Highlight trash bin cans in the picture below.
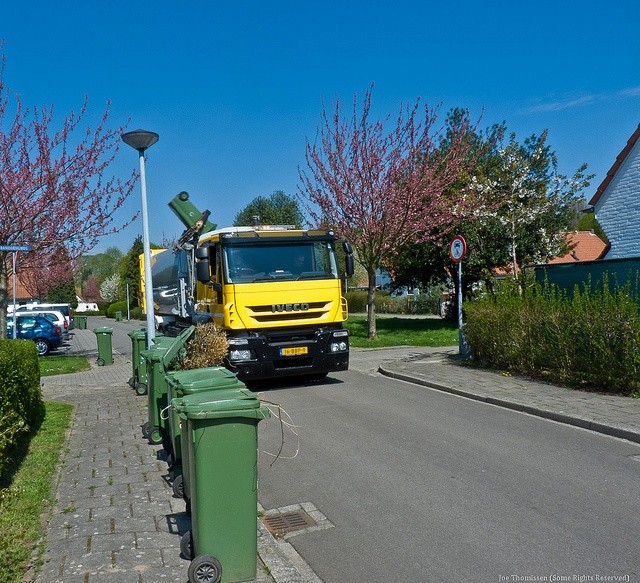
[139,344,169,444]
[73,316,80,328]
[169,387,271,583]
[115,311,122,322]
[127,328,165,394]
[79,316,87,329]
[163,364,246,497]
[167,191,217,236]
[152,336,190,424]
[161,325,220,448]
[93,326,114,365]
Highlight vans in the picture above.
[12,303,73,330]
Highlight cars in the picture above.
[6,316,63,356]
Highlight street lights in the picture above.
[122,128,159,349]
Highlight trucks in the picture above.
[138,216,354,389]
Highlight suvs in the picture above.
[7,311,68,350]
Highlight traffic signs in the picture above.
[0,245,30,251]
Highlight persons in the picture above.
[287,253,307,275]
